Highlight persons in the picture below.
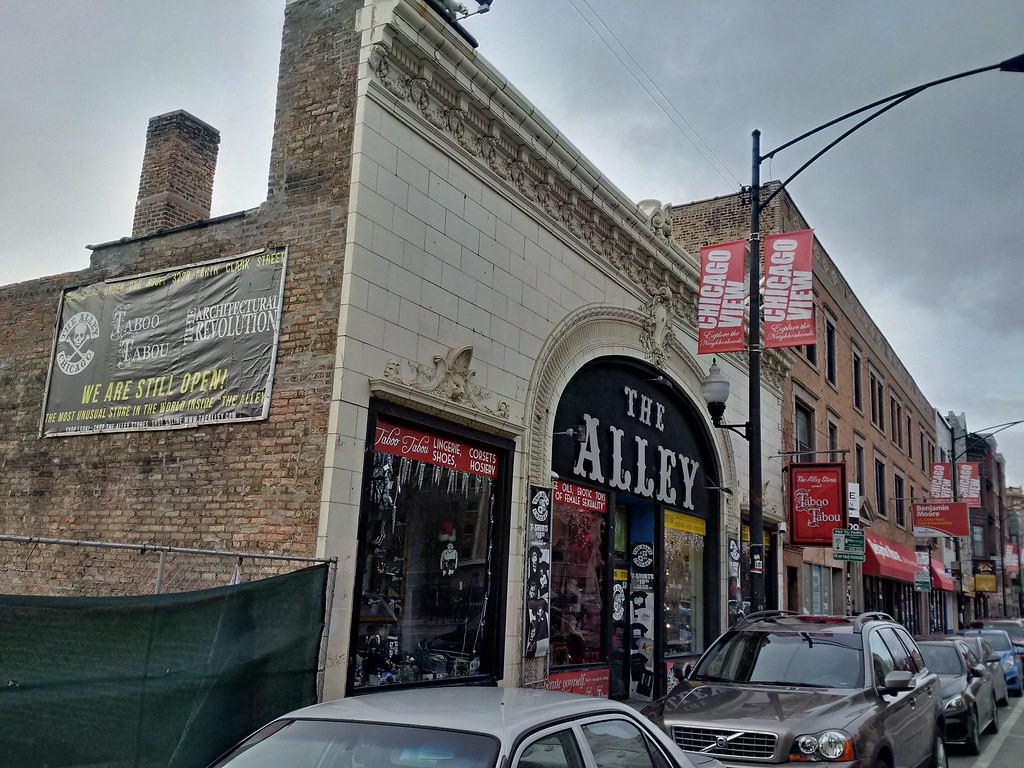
[550,577,587,665]
[728,576,737,600]
[526,545,548,657]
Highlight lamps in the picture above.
[552,425,586,442]
[705,487,734,498]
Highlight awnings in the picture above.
[931,562,954,592]
[864,531,925,583]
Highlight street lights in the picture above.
[700,48,1024,611]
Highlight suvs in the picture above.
[635,610,951,768]
[960,616,1024,664]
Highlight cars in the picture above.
[911,637,1000,757]
[923,634,1010,709]
[955,628,1024,698]
[205,683,720,767]
